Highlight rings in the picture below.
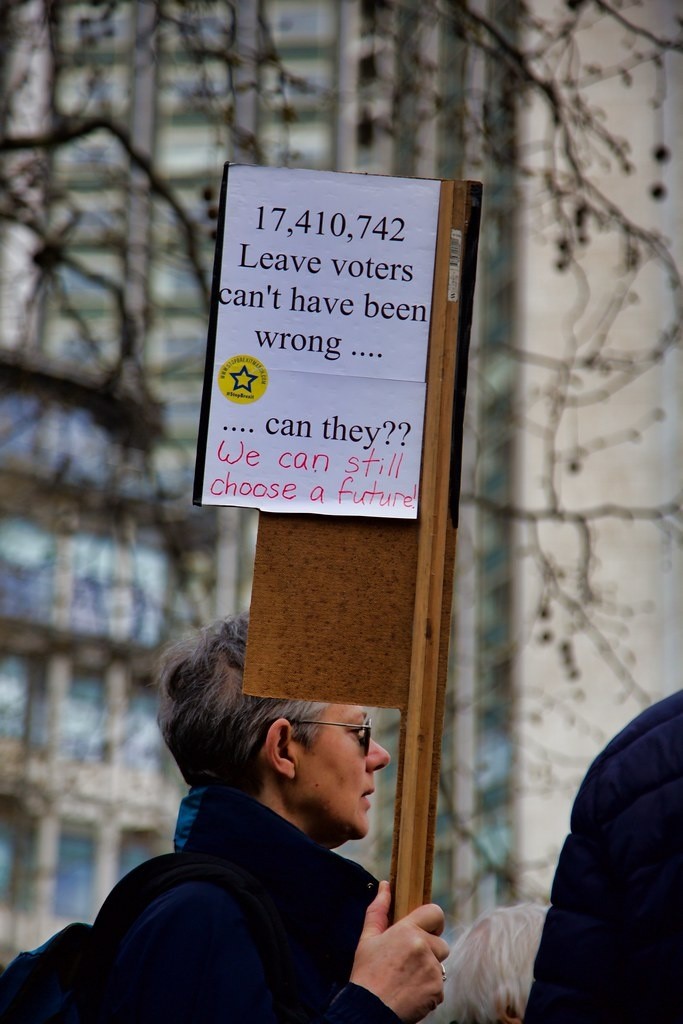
[441,963,446,981]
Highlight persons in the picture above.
[66,618,450,1024]
[442,903,552,1024]
[519,689,683,1024]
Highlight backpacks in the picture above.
[0,851,301,1024]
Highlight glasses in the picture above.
[249,716,373,770]
